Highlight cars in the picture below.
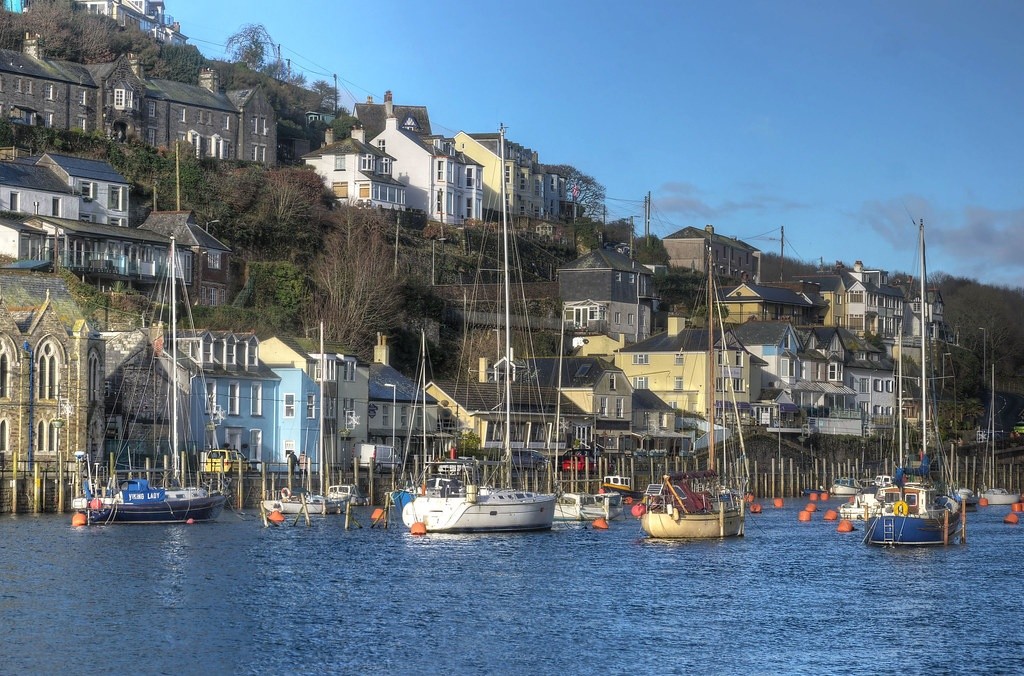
[562,455,599,474]
[203,450,249,472]
[501,449,550,471]
[551,449,594,471]
[438,459,478,477]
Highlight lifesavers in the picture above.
[893,500,909,516]
[281,486,291,499]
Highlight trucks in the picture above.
[350,442,404,473]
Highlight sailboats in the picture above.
[640,246,749,538]
[390,327,466,511]
[71,235,231,526]
[540,312,625,521]
[261,317,349,513]
[863,217,965,547]
[976,362,1019,504]
[401,120,557,535]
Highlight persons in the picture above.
[298,451,309,479]
[287,451,298,476]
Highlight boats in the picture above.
[831,475,979,520]
[327,483,366,507]
